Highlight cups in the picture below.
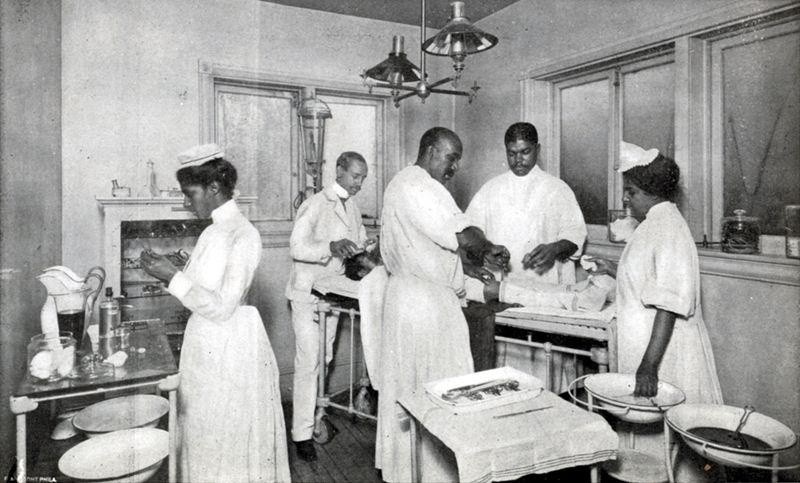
[50,291,88,358]
[783,205,800,260]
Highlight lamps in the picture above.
[360,1,499,109]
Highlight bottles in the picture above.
[25,330,77,384]
[608,209,640,245]
[98,287,122,341]
[720,209,762,255]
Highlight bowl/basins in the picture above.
[58,427,172,483]
[51,417,79,441]
[582,372,687,422]
[666,403,797,468]
[71,393,174,438]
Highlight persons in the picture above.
[466,122,588,397]
[341,243,608,312]
[284,151,369,464]
[375,125,509,482]
[581,141,727,419]
[138,140,292,483]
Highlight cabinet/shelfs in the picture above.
[97,194,256,351]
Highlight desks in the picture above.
[8,320,182,481]
[396,369,616,482]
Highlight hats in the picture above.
[176,144,223,171]
[618,141,659,173]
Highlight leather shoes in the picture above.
[293,439,316,462]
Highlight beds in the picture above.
[311,274,618,446]
